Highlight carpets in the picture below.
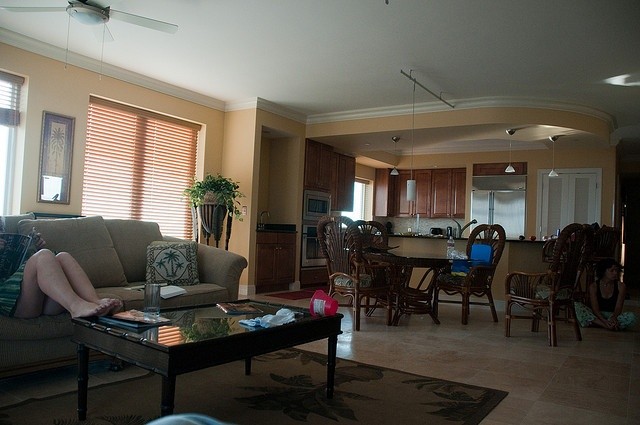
[0,346,510,424]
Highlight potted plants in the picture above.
[179,171,244,223]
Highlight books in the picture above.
[0,231,49,280]
[216,301,264,315]
[106,309,172,323]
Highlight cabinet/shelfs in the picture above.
[431,169,465,218]
[331,151,356,211]
[394,169,431,218]
[300,270,328,288]
[256,232,297,287]
[375,167,396,218]
[304,138,334,191]
[536,167,603,242]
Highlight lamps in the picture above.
[504,165,516,173]
[390,167,400,176]
[549,170,559,176]
[406,179,416,202]
[73,11,101,27]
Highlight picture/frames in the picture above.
[35,108,77,205]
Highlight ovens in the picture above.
[300,224,327,271]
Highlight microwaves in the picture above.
[303,189,332,221]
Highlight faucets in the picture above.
[453,220,477,238]
[258,211,270,231]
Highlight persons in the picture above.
[0,216,124,322]
[572,257,639,332]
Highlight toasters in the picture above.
[429,228,443,237]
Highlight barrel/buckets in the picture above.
[309,290,339,317]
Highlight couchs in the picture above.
[0,236,250,381]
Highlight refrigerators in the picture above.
[470,190,527,240]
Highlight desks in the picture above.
[398,254,463,294]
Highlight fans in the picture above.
[0,0,178,35]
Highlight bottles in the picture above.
[447,236,455,259]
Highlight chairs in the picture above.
[344,218,397,319]
[363,248,442,326]
[503,222,590,348]
[593,224,620,259]
[541,223,592,263]
[432,222,501,326]
[316,214,393,331]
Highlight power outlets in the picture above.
[408,228,412,232]
[242,206,247,215]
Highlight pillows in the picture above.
[104,218,163,282]
[17,215,129,289]
[0,212,36,235]
[145,239,200,286]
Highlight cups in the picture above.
[144,284,160,316]
